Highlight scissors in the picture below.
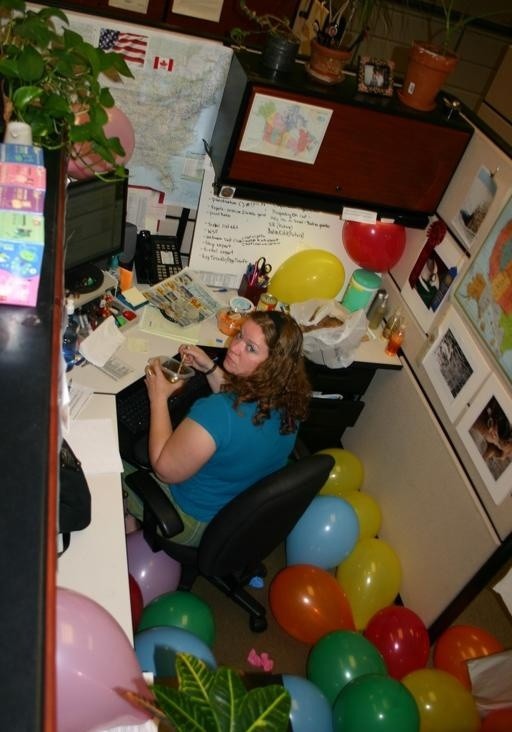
[257,257,271,274]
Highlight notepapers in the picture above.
[121,287,148,306]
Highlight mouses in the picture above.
[206,351,219,362]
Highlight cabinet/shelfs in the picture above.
[205,42,475,232]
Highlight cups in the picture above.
[3,119,33,145]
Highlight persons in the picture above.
[121,310,313,549]
[369,71,384,88]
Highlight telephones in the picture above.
[135,230,182,283]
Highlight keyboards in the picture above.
[116,369,204,435]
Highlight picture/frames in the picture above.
[448,180,512,397]
[418,302,493,428]
[355,54,398,95]
[455,369,512,508]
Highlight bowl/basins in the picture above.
[65,262,105,294]
[215,307,248,337]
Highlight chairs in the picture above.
[123,415,337,634]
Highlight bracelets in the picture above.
[204,362,217,376]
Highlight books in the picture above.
[139,305,201,346]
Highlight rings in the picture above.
[147,370,153,376]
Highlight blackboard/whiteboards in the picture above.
[189,169,382,304]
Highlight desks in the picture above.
[45,260,402,465]
[54,381,157,732]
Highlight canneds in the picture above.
[256,292,278,311]
[216,297,255,337]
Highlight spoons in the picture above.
[170,347,193,385]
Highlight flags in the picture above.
[98,27,149,67]
[152,56,173,72]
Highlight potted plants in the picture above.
[301,0,394,87]
[395,0,510,113]
[226,0,302,87]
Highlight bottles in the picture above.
[383,306,406,356]
[341,269,382,315]
[366,289,389,331]
[62,304,78,374]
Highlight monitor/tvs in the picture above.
[64,167,129,294]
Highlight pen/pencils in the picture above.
[247,263,271,287]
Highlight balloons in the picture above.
[282,605,479,732]
[269,446,402,643]
[55,586,157,732]
[341,219,406,273]
[67,101,136,181]
[127,530,216,688]
[267,248,346,306]
[436,623,512,732]
